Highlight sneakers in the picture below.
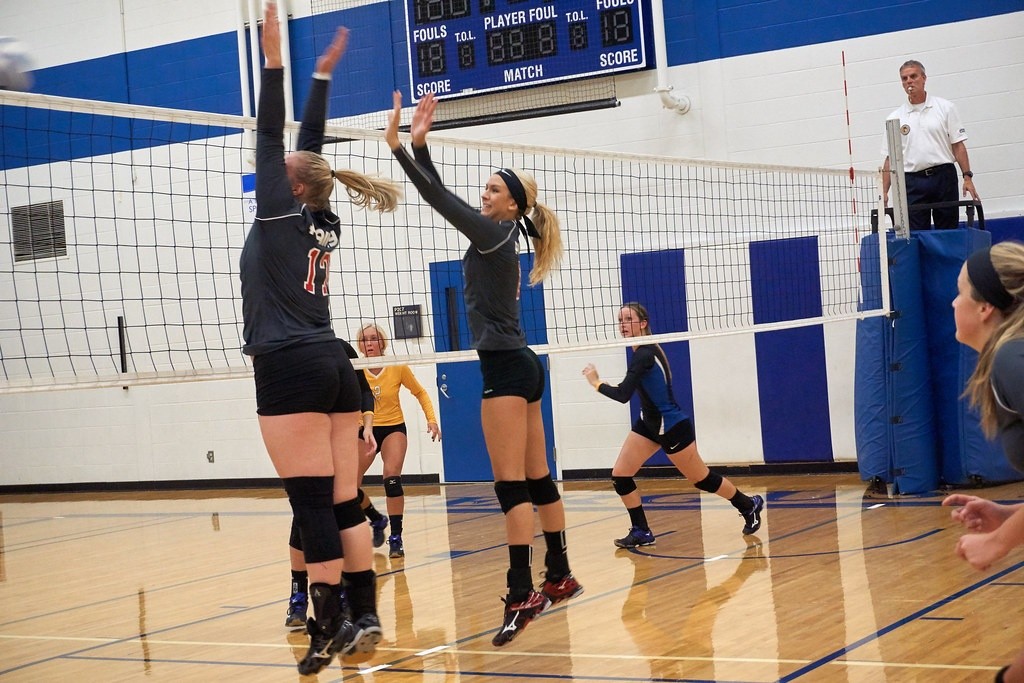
[284,592,309,627]
[347,613,384,656]
[491,588,553,648]
[370,515,388,548]
[614,526,657,549]
[296,614,365,677]
[740,494,764,536]
[534,569,585,609]
[385,534,405,558]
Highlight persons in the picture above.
[942,242,1024,683]
[284,337,377,627]
[239,0,384,676]
[385,90,585,646]
[357,323,442,558]
[882,60,981,231]
[582,302,764,548]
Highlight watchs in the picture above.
[963,171,973,178]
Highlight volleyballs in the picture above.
[0,36,35,91]
